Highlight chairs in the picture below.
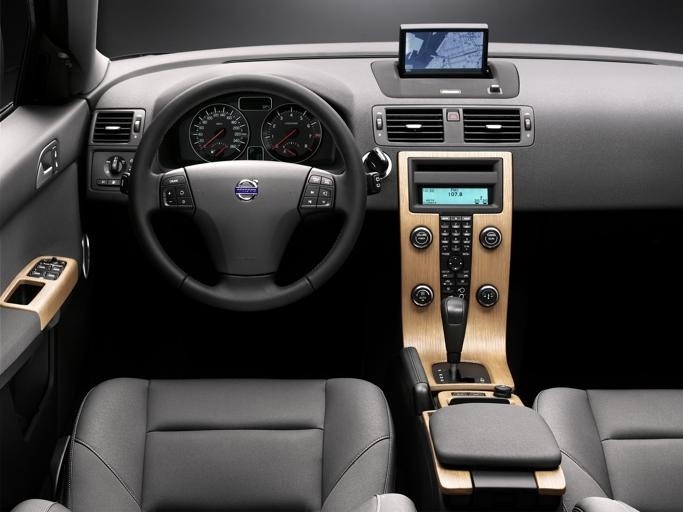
[532,386,682,511]
[8,376,417,512]
